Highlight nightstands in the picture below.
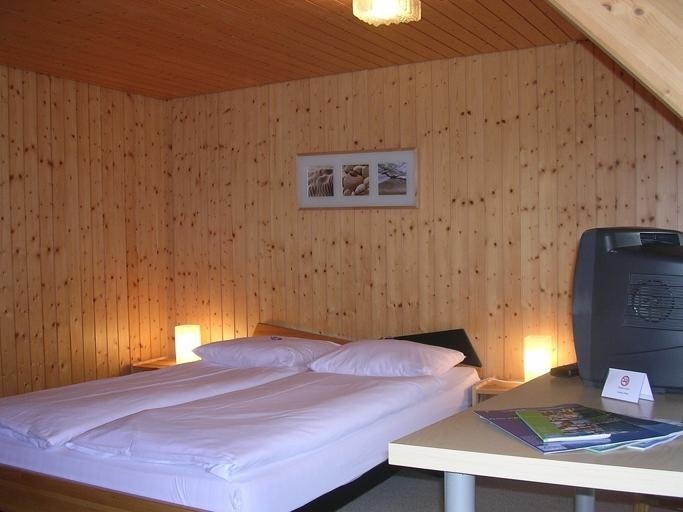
[470,376,523,407]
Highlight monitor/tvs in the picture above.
[572,226,683,395]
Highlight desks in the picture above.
[387,365,682,512]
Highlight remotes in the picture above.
[551,363,579,378]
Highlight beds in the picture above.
[0,322,480,512]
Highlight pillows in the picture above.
[190,334,465,378]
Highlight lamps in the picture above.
[174,324,200,365]
[353,0,421,28]
[524,335,551,383]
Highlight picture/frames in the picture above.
[294,146,418,209]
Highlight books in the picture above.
[472,403,683,456]
